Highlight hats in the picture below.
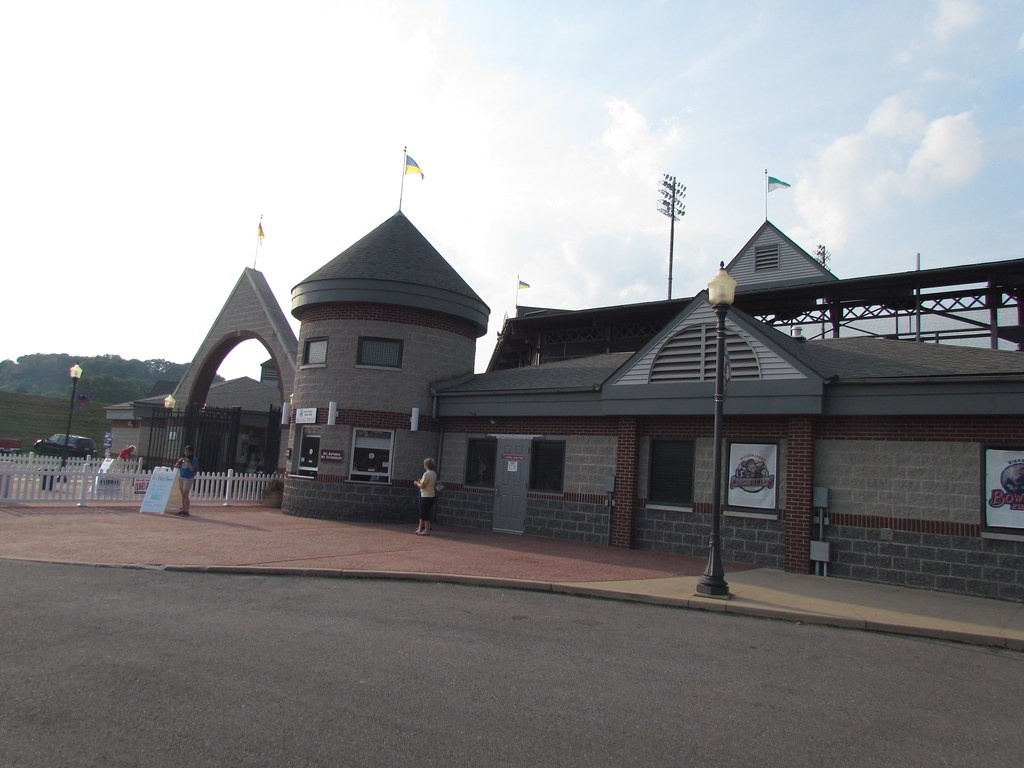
[185,446,193,452]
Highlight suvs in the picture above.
[33,433,98,462]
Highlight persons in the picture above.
[175,445,199,517]
[254,461,264,475]
[414,457,439,537]
[117,445,136,463]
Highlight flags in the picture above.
[518,281,530,289]
[768,177,790,192]
[258,223,265,246]
[405,155,425,180]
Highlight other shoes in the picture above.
[418,532,430,536]
[414,527,424,531]
[422,527,432,530]
[414,530,420,534]
[176,511,184,515]
[181,512,189,517]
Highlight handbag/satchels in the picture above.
[435,479,445,492]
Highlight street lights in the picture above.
[693,260,736,601]
[159,392,177,467]
[54,360,83,483]
